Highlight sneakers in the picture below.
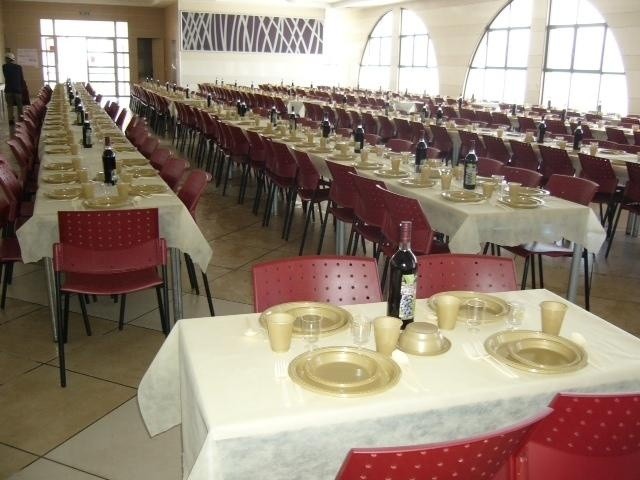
[9,120,15,125]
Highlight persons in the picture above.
[2,52,30,126]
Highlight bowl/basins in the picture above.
[401,322,444,351]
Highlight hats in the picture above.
[3,53,16,61]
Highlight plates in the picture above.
[509,339,580,366]
[285,306,343,331]
[373,170,408,178]
[400,336,450,356]
[136,184,166,194]
[290,346,400,396]
[46,84,65,121]
[45,133,66,137]
[133,168,158,176]
[499,195,544,208]
[601,149,624,154]
[486,331,586,373]
[351,162,383,169]
[43,162,71,169]
[45,121,62,125]
[259,301,352,338]
[427,291,509,320]
[125,158,150,165]
[297,142,317,147]
[92,196,126,207]
[44,149,68,153]
[442,190,485,202]
[42,174,77,183]
[310,148,335,153]
[613,160,626,165]
[45,189,82,198]
[43,126,63,130]
[117,147,134,151]
[306,351,378,388]
[522,187,550,196]
[44,140,66,144]
[400,178,435,187]
[329,154,356,160]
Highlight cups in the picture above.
[450,120,455,128]
[596,122,602,128]
[64,82,74,144]
[116,183,129,198]
[71,144,79,155]
[590,142,598,146]
[401,152,410,163]
[468,299,484,327]
[590,146,597,156]
[360,149,369,162]
[116,160,122,171]
[441,175,451,190]
[375,145,385,157]
[122,174,131,182]
[307,134,313,143]
[483,183,495,199]
[436,295,457,328]
[373,316,403,355]
[493,175,503,191]
[227,112,230,119]
[510,303,522,324]
[78,170,87,182]
[349,315,370,344]
[390,156,401,175]
[256,118,260,125]
[508,182,522,199]
[560,140,566,149]
[266,313,292,350]
[525,132,532,143]
[540,300,567,335]
[71,158,80,169]
[301,314,320,341]
[472,123,480,130]
[497,129,503,138]
[220,84,300,101]
[82,182,94,197]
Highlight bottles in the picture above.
[216,77,314,91]
[418,88,603,121]
[241,100,245,116]
[435,103,443,125]
[388,220,416,328]
[237,97,241,114]
[323,112,331,136]
[421,106,427,124]
[538,115,546,143]
[464,141,476,188]
[146,76,212,107]
[354,114,365,153]
[289,106,296,135]
[271,99,277,126]
[103,136,117,184]
[84,111,93,148]
[333,84,409,98]
[416,130,428,173]
[67,79,86,124]
[573,121,582,151]
